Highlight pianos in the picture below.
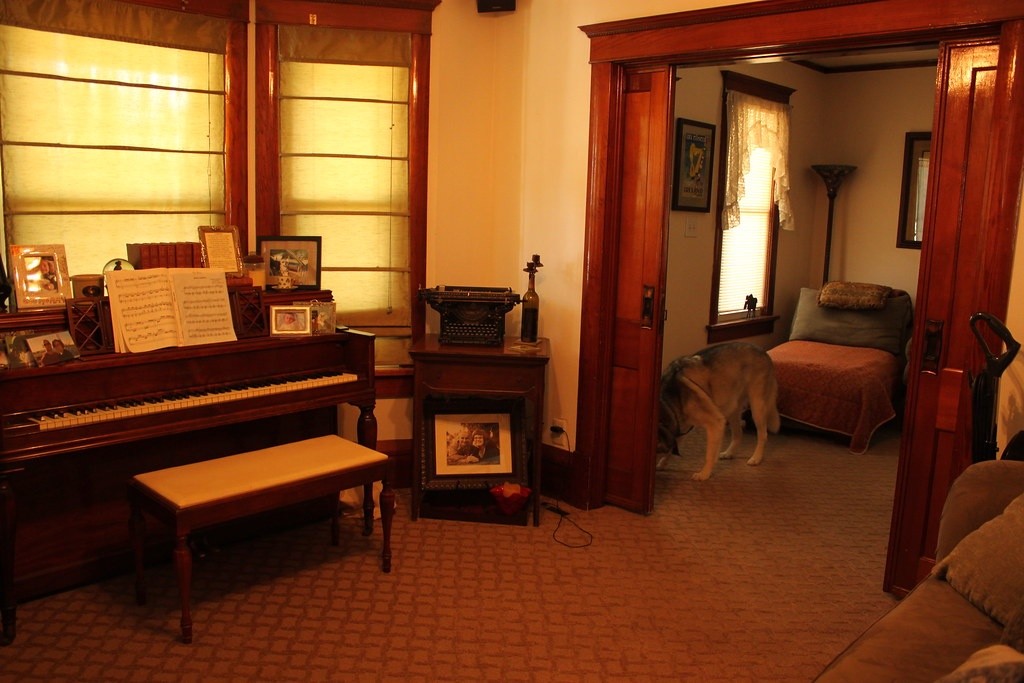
[2,285,378,645]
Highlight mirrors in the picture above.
[895,130,932,249]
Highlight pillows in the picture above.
[932,490,1024,631]
[818,281,888,312]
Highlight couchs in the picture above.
[808,461,1024,683]
[751,285,913,455]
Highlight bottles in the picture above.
[521,275,539,343]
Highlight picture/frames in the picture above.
[673,117,717,213]
[0,226,513,475]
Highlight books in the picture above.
[105,267,237,353]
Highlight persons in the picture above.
[280,313,301,330]
[40,339,74,367]
[40,258,56,289]
[9,341,34,365]
[448,429,498,463]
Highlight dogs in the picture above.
[657,341,780,481]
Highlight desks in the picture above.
[408,333,552,527]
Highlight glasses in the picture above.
[44,342,50,346]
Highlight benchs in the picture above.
[132,436,394,643]
